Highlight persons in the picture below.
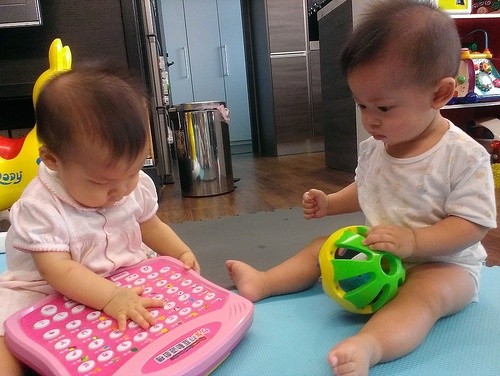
[1,57,202,376]
[218,0,497,375]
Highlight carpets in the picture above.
[205,264,500,376]
[167,204,372,292]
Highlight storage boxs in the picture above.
[420,0,472,14]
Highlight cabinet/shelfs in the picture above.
[251,0,324,157]
[442,12,500,109]
[160,0,254,154]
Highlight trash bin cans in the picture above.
[175,101,241,199]
